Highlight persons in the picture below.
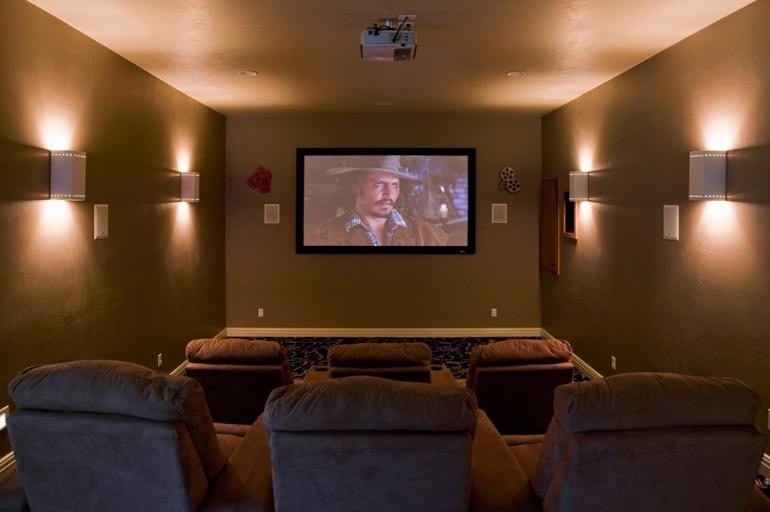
[309,155,449,246]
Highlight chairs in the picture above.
[504,372,769,511]
[465,337,574,435]
[6,360,274,511]
[327,342,432,379]
[185,338,301,424]
[264,363,477,510]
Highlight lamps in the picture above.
[688,151,728,200]
[49,151,86,203]
[180,172,201,202]
[568,171,588,201]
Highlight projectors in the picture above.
[360,31,418,61]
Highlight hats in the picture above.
[326,156,423,186]
